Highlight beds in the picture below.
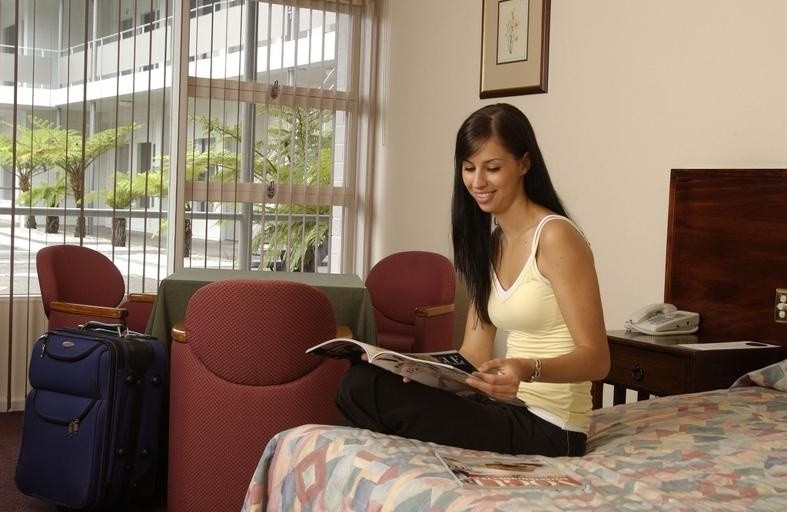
[240,170,785,512]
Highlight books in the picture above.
[305,337,483,394]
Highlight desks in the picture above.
[143,268,377,344]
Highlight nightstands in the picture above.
[589,327,780,409]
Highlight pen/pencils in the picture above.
[746,343,768,346]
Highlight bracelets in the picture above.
[525,357,541,382]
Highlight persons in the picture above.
[336,103,612,456]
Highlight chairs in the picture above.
[166,279,354,510]
[363,250,455,352]
[36,245,158,335]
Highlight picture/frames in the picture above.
[477,1,549,100]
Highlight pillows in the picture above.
[728,359,785,392]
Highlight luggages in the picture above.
[14,320,169,512]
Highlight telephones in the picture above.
[624,302,700,336]
